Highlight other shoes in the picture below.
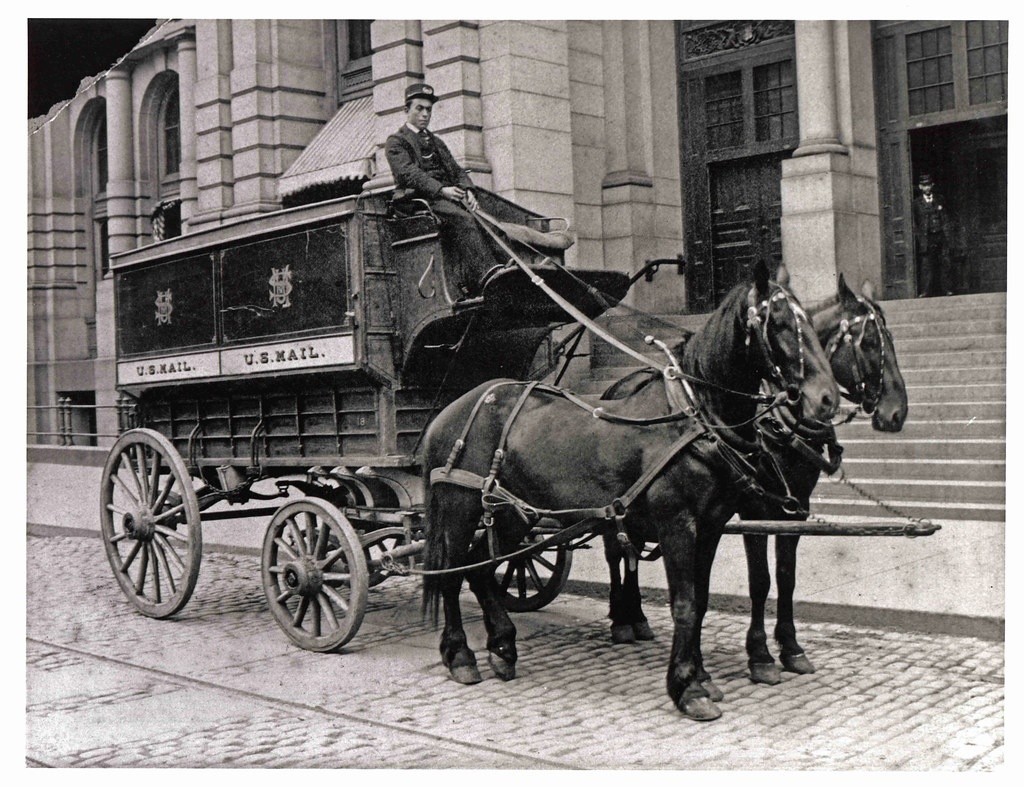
[919,292,931,298]
[946,291,954,296]
[478,263,505,288]
[532,255,562,267]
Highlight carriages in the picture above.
[96,183,947,727]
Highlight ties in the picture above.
[419,130,427,138]
[927,197,932,206]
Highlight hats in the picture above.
[918,174,933,185]
[405,84,439,104]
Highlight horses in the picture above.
[420,260,908,721]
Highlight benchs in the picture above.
[385,187,570,345]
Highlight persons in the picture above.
[911,173,958,299]
[383,82,517,297]
